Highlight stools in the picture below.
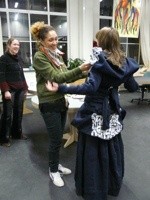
[131,83,150,105]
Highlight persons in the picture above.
[31,21,91,187]
[45,26,140,200]
[0,38,28,146]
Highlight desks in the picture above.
[31,95,87,149]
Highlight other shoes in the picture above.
[7,139,13,146]
[13,134,28,139]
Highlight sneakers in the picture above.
[49,167,65,186]
[58,163,71,174]
